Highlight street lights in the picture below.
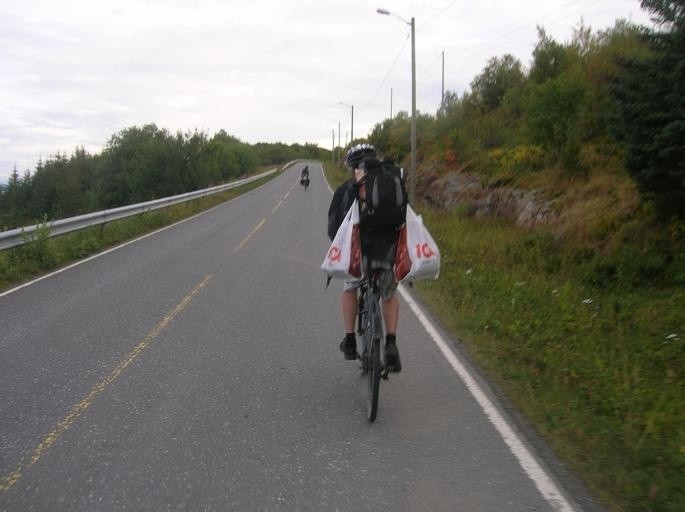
[338,102,354,147]
[377,9,417,208]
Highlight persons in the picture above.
[327,142,412,375]
[301,164,309,183]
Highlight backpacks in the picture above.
[355,172,407,262]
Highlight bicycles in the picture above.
[300,174,310,191]
[344,264,401,423]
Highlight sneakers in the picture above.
[339,333,356,360]
[383,335,401,374]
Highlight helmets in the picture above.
[346,143,375,166]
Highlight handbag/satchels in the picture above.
[393,203,441,284]
[320,199,364,282]
